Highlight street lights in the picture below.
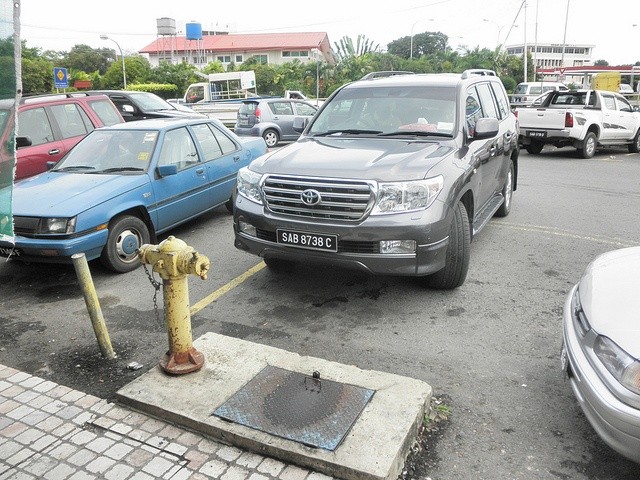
[99,33,131,91]
[480,14,518,61]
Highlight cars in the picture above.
[0,117,263,274]
[561,244,640,466]
[234,98,318,149]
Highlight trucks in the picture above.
[183,82,247,104]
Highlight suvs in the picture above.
[233,68,520,291]
[79,87,211,122]
[0,95,128,191]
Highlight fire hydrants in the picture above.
[134,235,211,375]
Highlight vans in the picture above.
[512,81,570,108]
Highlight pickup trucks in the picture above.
[517,90,640,158]
